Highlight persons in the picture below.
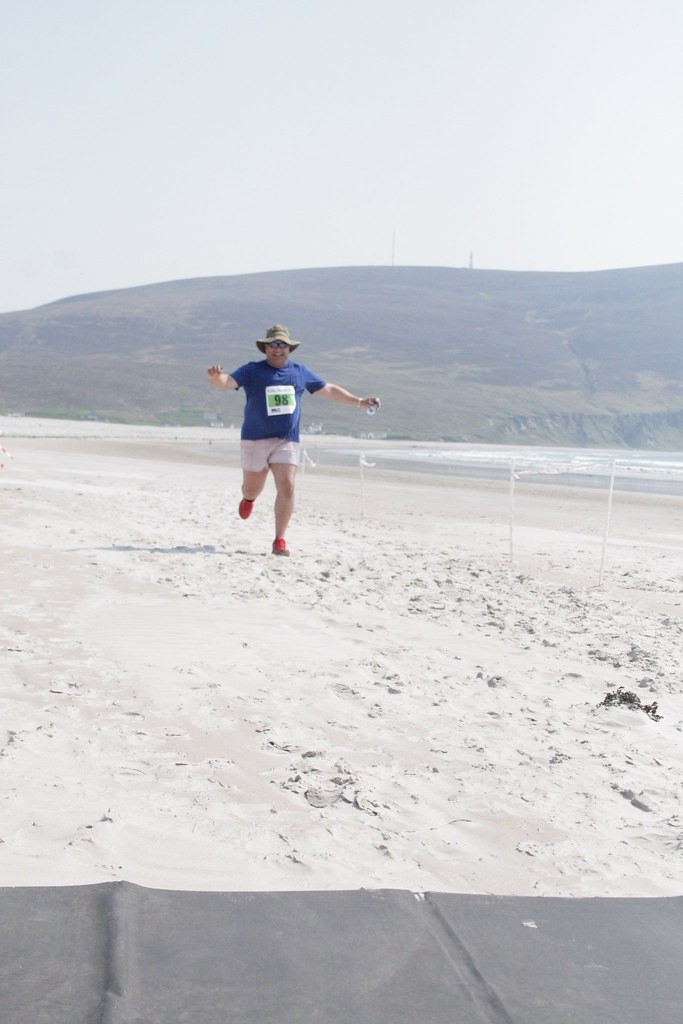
[208,325,381,556]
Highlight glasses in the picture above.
[266,342,289,349]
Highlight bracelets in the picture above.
[357,397,363,408]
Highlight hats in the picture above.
[256,325,301,353]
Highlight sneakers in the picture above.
[238,498,254,519]
[272,536,290,556]
